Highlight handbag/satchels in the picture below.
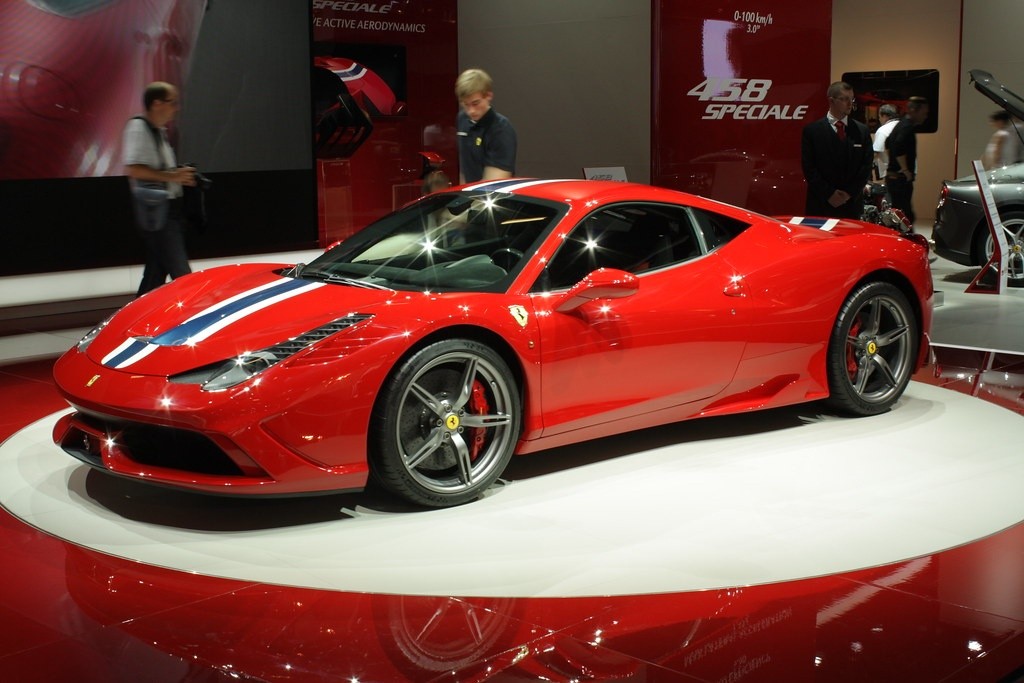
[131,186,170,232]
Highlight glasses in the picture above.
[834,97,856,102]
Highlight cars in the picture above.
[929,67,1024,285]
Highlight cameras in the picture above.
[178,162,212,189]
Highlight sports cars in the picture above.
[50,176,935,509]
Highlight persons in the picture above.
[979,109,1024,171]
[453,68,518,244]
[798,81,873,221]
[122,81,195,299]
[871,103,919,233]
[421,170,449,195]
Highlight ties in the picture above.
[835,121,845,141]
